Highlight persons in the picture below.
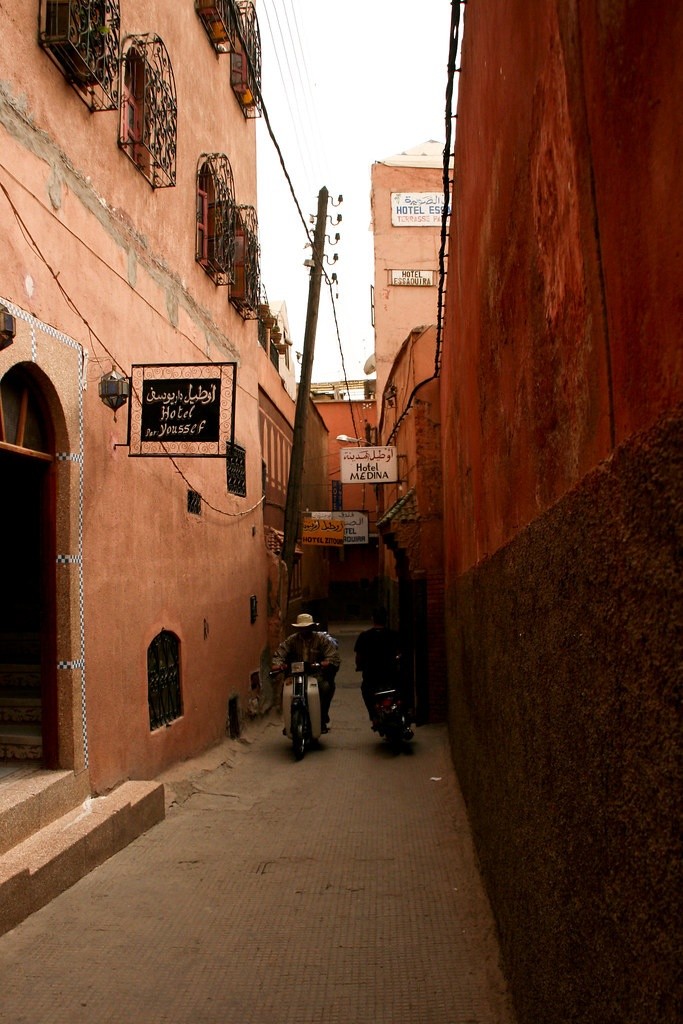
[354,606,416,732]
[272,613,342,736]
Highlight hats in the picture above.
[291,613,320,627]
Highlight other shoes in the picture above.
[321,722,328,735]
[283,727,288,735]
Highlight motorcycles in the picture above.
[356,657,415,755]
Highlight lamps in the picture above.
[98,364,133,450]
[336,434,375,447]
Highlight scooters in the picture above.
[264,660,338,761]
